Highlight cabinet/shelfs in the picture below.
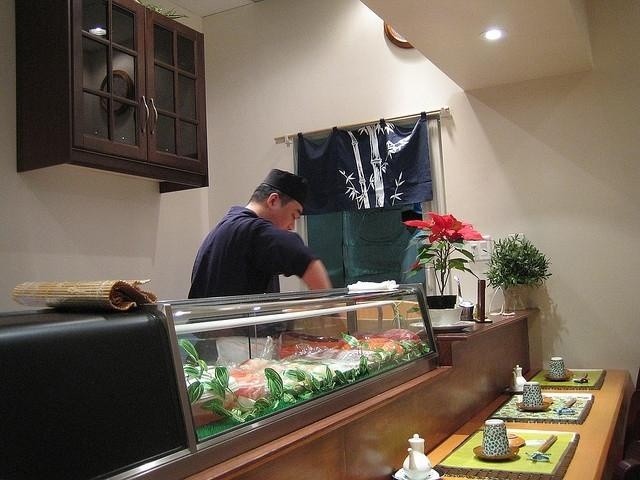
[16,0,209,194]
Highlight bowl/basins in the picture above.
[426,306,462,326]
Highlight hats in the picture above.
[264,169,309,207]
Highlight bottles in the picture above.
[459,301,475,320]
[408,433,425,452]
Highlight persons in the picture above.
[179,168,347,365]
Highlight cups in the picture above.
[482,419,510,456]
[549,358,565,379]
[522,383,544,407]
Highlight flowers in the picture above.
[401,211,486,294]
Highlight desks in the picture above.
[389,367,633,480]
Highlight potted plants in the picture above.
[482,232,554,312]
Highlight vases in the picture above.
[426,295,457,310]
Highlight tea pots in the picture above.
[511,365,528,392]
[403,448,432,478]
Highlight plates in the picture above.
[546,370,574,381]
[516,401,550,411]
[393,466,440,480]
[473,445,519,460]
[409,319,476,334]
